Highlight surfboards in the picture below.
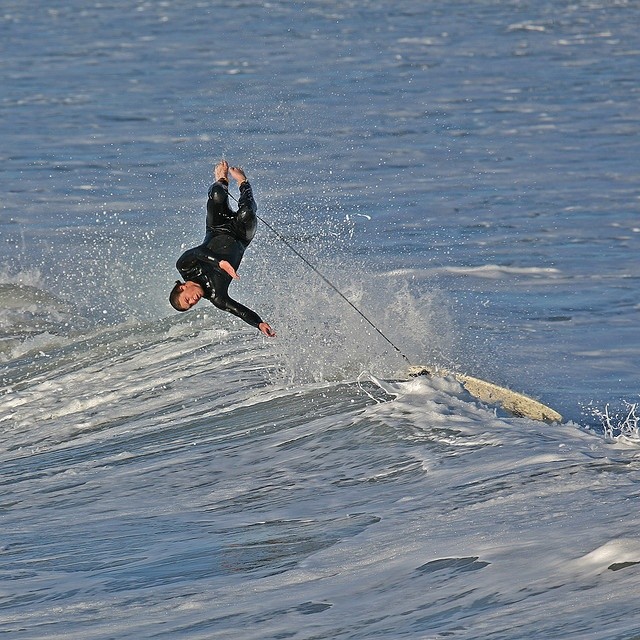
[405,364,563,430]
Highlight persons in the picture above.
[169,161,275,337]
